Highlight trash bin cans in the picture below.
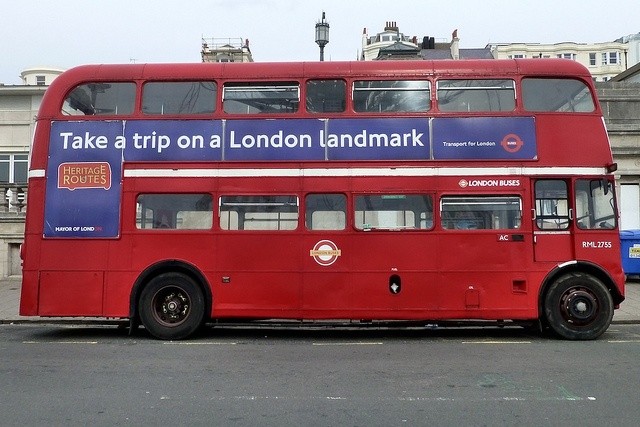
[620,230,640,280]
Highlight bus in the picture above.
[19,59,625,339]
